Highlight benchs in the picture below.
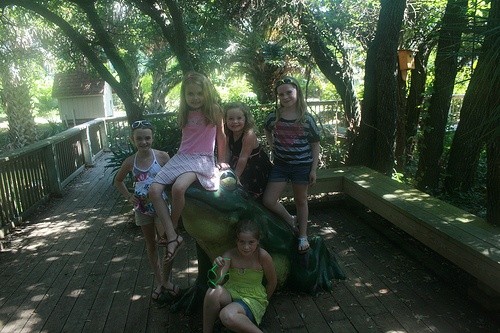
[342,164,500,290]
[281,166,349,195]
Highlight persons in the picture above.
[202,218,277,333]
[224,102,273,203]
[262,76,320,251]
[147,72,226,261]
[115,120,181,303]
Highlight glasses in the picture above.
[132,120,152,129]
[275,78,292,87]
[206,257,231,289]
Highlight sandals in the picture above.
[161,282,182,300]
[164,233,185,264]
[290,215,299,237]
[296,234,311,254]
[151,286,163,302]
[158,232,167,245]
